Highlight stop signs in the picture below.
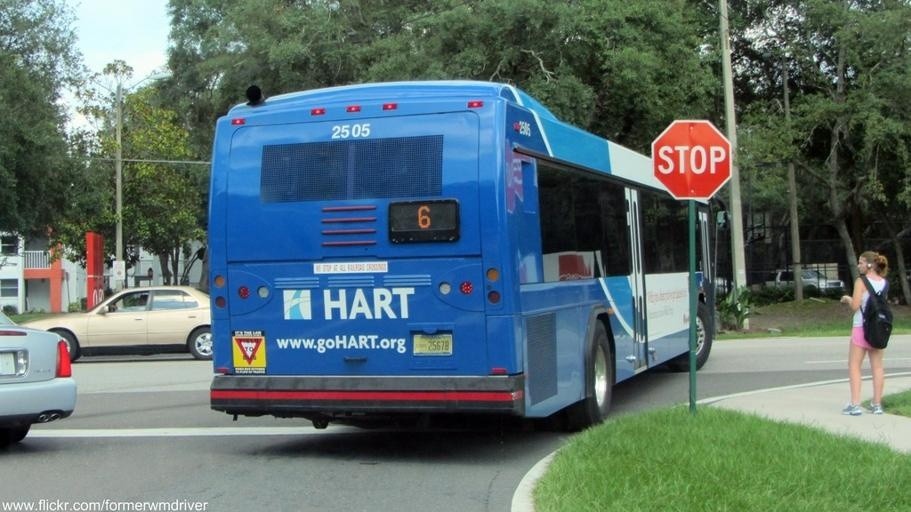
[646,115,734,206]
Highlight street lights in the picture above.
[95,53,138,294]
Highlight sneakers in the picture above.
[867,400,883,415]
[843,404,861,416]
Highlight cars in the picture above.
[715,270,846,298]
[0,302,82,450]
[18,282,215,360]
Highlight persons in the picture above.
[839,250,891,415]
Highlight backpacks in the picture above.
[855,274,893,349]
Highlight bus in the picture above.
[194,79,714,429]
